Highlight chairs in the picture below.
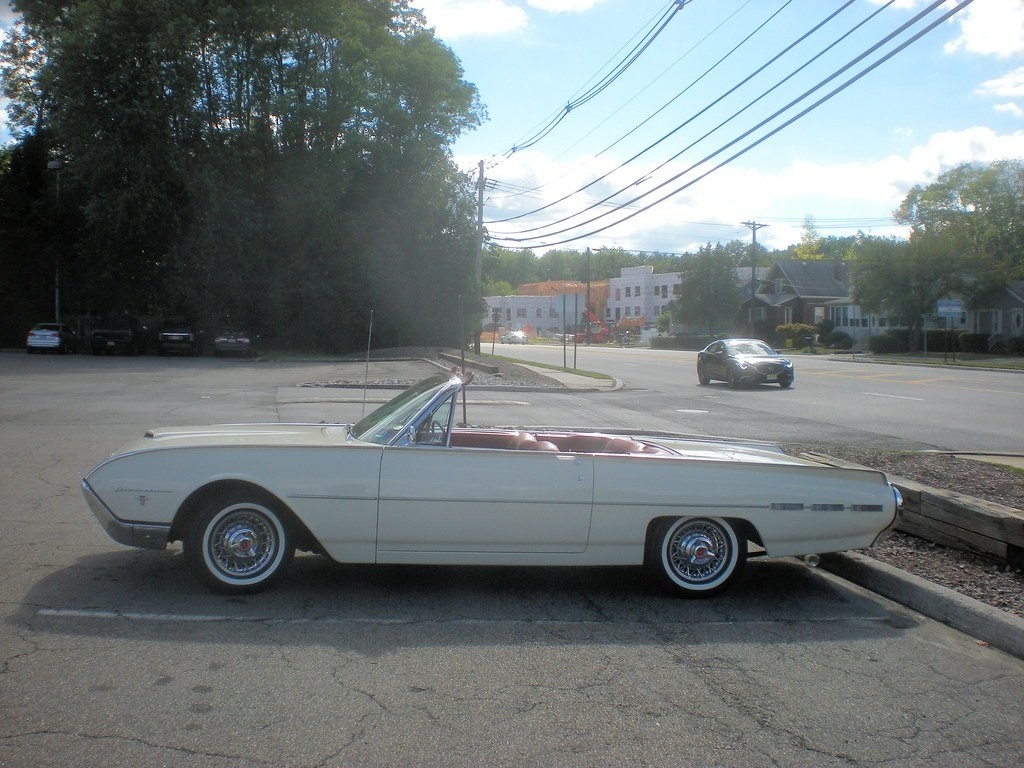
[509,432,645,456]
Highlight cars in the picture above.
[214,330,250,358]
[90,313,151,358]
[158,328,200,356]
[78,369,907,596]
[696,337,795,390]
[26,321,77,353]
[501,331,527,345]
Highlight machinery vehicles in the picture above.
[572,306,644,345]
